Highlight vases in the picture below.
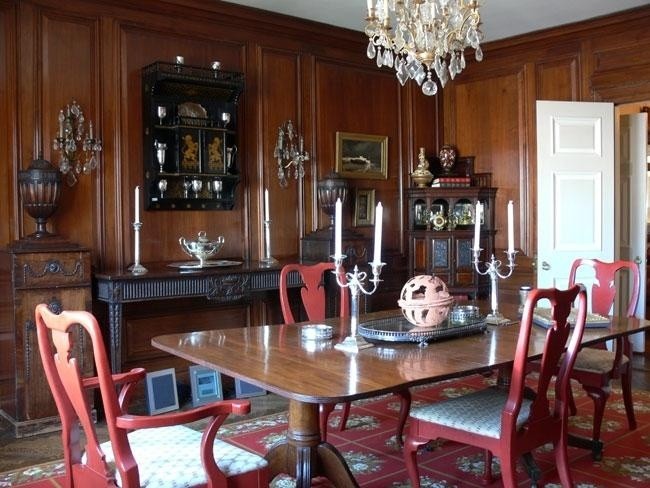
[412,146,433,188]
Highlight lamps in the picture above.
[50,96,104,189]
[365,0,485,97]
[275,119,312,190]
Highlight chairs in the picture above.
[33,302,271,488]
[279,263,410,447]
[403,284,588,488]
[532,258,640,462]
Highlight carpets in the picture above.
[0,366,649,488]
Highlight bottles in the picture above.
[519,285,531,305]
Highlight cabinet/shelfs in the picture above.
[405,185,497,300]
[141,61,246,213]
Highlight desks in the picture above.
[100,258,327,397]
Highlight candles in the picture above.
[58,110,64,138]
[133,184,140,222]
[507,197,514,251]
[264,187,271,224]
[89,120,92,139]
[473,199,481,250]
[372,200,383,264]
[334,195,342,257]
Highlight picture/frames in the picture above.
[335,131,389,181]
[353,187,376,227]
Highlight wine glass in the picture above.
[192,178,203,198]
[213,179,223,199]
[222,111,231,127]
[159,179,167,198]
[209,60,222,78]
[157,106,167,125]
[175,55,185,73]
